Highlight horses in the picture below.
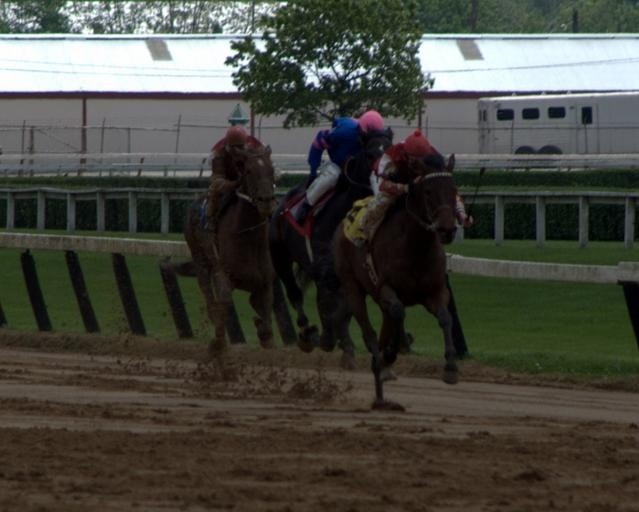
[328,152,469,386]
[269,124,395,364]
[185,145,278,380]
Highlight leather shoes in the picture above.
[295,196,314,227]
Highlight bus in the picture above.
[477,92,639,154]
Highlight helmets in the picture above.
[358,110,385,133]
[403,130,431,156]
[224,126,248,145]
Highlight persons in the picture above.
[194,126,267,237]
[293,109,396,227]
[348,125,476,251]
